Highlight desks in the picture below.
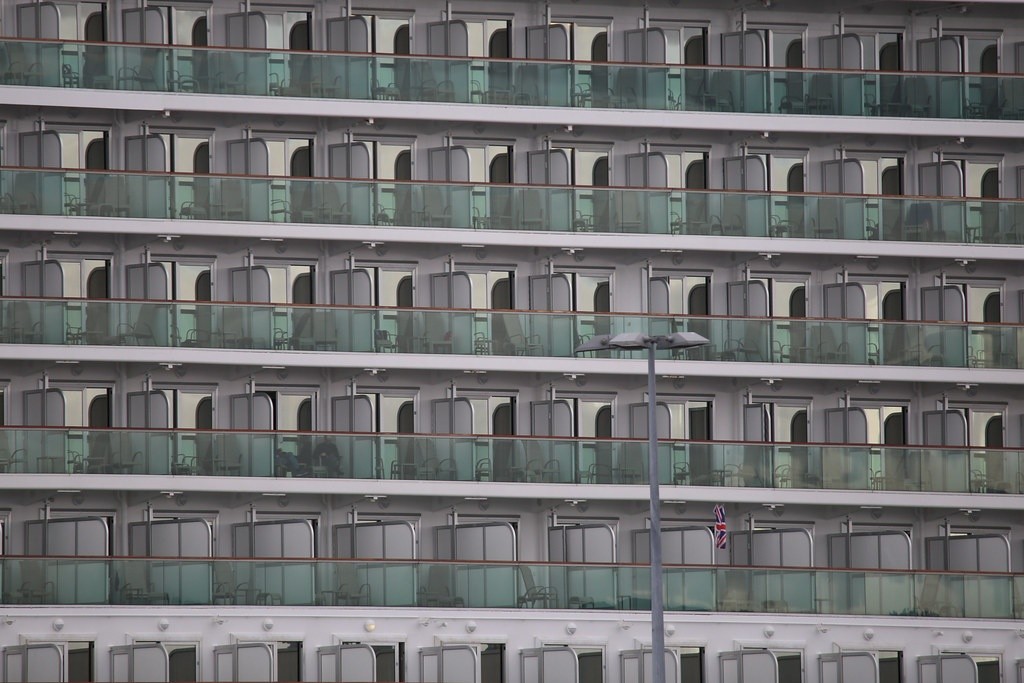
[83,457,107,475]
[309,465,328,478]
[789,348,815,364]
[807,97,831,115]
[712,470,732,487]
[289,337,316,350]
[400,87,436,102]
[93,75,114,90]
[298,81,323,98]
[486,88,511,104]
[904,225,930,242]
[692,94,718,111]
[394,463,419,480]
[883,102,911,117]
[601,96,628,109]
[194,76,220,94]
[5,72,39,86]
[303,208,331,224]
[494,216,514,229]
[81,331,106,345]
[209,332,238,348]
[409,337,432,354]
[399,211,426,227]
[682,221,710,235]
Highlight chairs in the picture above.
[0,59,1023,610]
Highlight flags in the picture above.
[714,503,727,549]
